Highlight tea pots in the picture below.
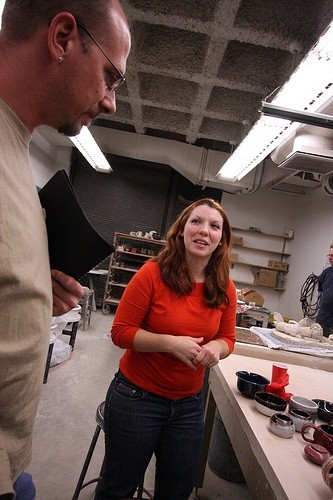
[321,454,333,493]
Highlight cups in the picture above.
[269,413,294,439]
[287,411,315,432]
[300,423,333,451]
[274,320,324,340]
[311,399,333,422]
[287,396,320,422]
[252,391,290,417]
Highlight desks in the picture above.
[42,304,82,384]
[194,353,333,500]
[87,269,109,312]
[236,307,271,328]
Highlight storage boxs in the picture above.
[231,253,239,263]
[241,287,265,307]
[268,259,289,271]
[251,267,279,288]
[231,235,244,247]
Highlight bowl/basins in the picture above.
[152,233,163,240]
[235,370,270,400]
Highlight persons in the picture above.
[315,244,333,338]
[94,198,236,500]
[0,0,131,500]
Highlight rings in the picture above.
[208,362,211,365]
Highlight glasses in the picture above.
[48,15,126,93]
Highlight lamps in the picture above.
[213,16,333,183]
[68,125,113,174]
[258,85,333,130]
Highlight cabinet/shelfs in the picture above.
[101,231,168,316]
[229,227,294,292]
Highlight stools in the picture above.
[71,401,154,500]
